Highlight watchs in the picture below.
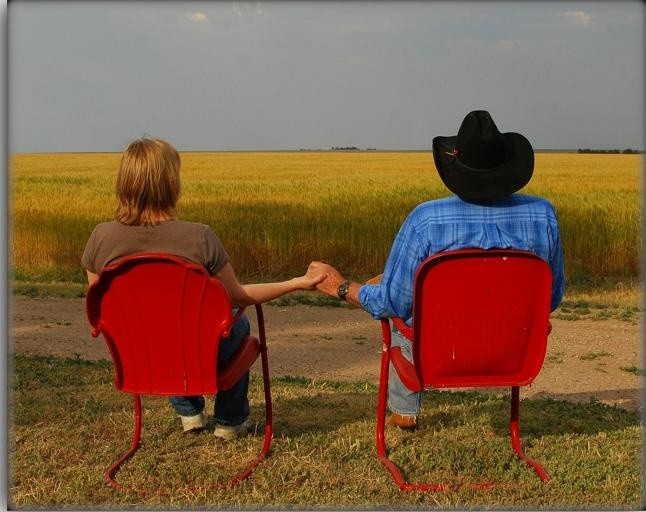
[338,278,351,302]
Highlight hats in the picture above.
[433,111,533,203]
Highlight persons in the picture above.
[82,139,328,443]
[310,109,565,431]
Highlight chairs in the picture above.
[376,248,551,494]
[85,251,272,496]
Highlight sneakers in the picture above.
[386,412,418,434]
[214,420,247,439]
[181,411,208,432]
